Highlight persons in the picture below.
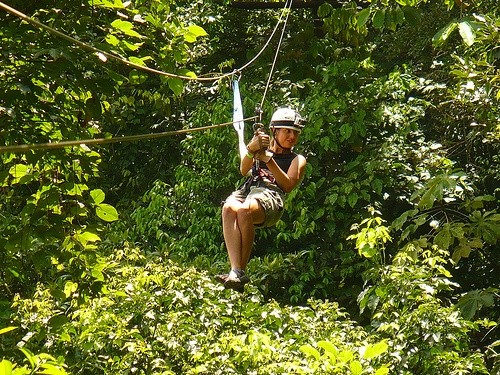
[214,108,306,294]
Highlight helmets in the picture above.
[269,108,309,135]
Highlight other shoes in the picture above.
[212,273,245,294]
[224,270,251,289]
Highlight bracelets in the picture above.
[246,145,255,158]
